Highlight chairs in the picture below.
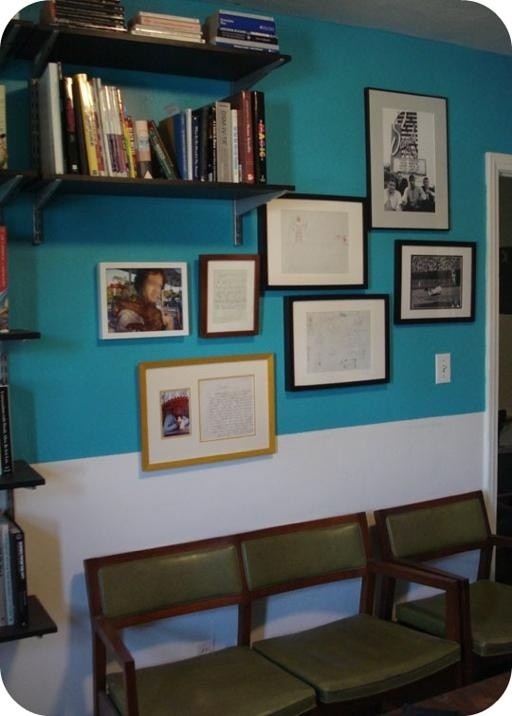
[373,486,511,677]
[79,532,321,716]
[234,510,467,711]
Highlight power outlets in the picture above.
[435,351,452,385]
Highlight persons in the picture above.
[396,170,408,194]
[402,175,426,210]
[163,405,188,433]
[113,267,174,330]
[422,177,436,211]
[384,177,402,210]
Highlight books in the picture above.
[1,84,33,636]
[42,0,278,53]
[37,62,266,183]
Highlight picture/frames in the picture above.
[392,235,476,325]
[94,256,191,343]
[279,291,392,395]
[195,250,261,339]
[361,84,451,231]
[256,189,370,292]
[136,354,277,473]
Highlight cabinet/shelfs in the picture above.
[0,168,57,644]
[21,22,296,248]
